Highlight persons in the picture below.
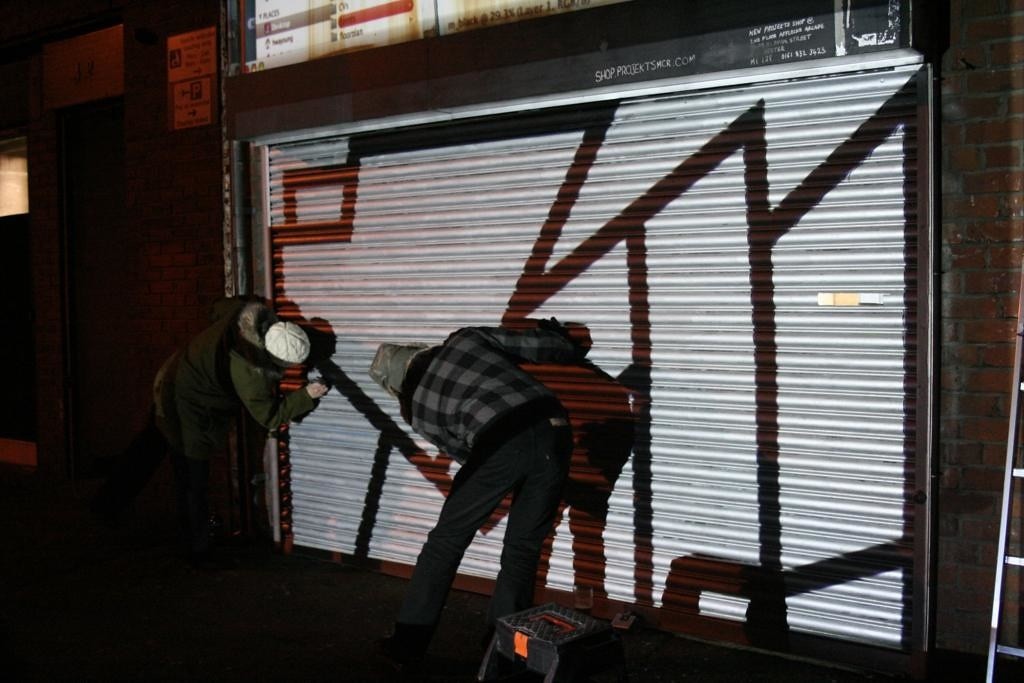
[152,294,327,550]
[368,326,577,664]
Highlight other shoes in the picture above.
[374,635,411,676]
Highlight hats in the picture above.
[264,321,310,362]
[370,342,423,390]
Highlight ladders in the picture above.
[985,257,1024,683]
